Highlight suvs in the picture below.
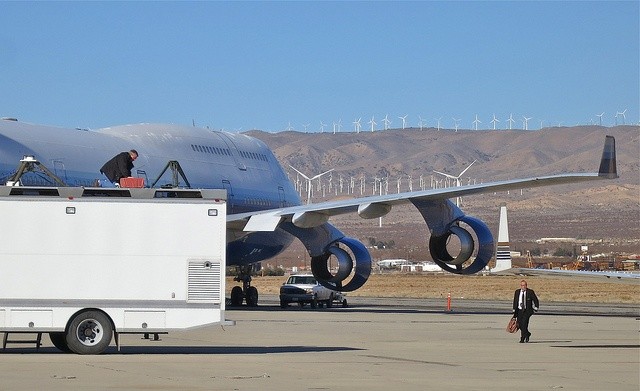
[280,275,335,308]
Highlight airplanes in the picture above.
[0,117,619,305]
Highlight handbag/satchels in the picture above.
[506,316,519,334]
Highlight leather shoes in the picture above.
[526,333,530,342]
[520,337,525,342]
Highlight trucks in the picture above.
[0,186,226,354]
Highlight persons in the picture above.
[513,281,539,344]
[99,150,138,187]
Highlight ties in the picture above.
[522,290,525,312]
